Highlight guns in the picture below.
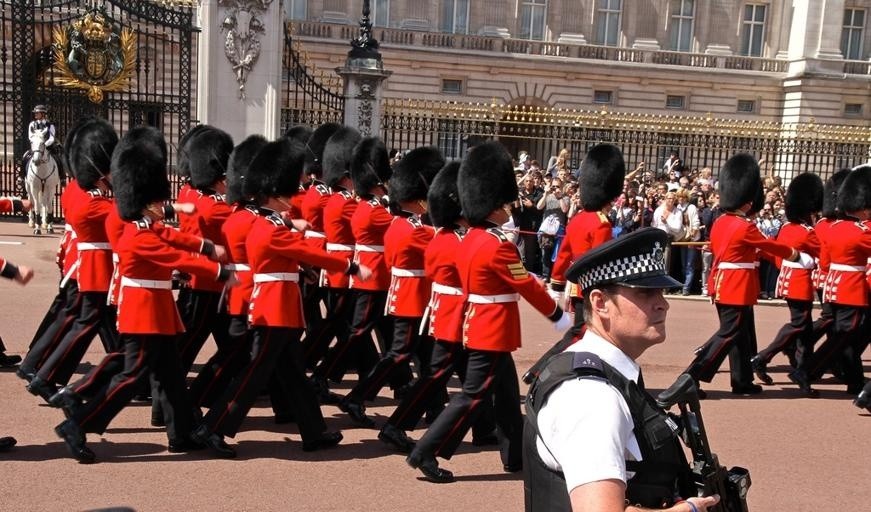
[658,372,752,512]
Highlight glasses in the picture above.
[552,186,559,188]
[676,161,679,163]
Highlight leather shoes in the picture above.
[788,369,820,397]
[694,346,718,373]
[693,381,708,399]
[53,419,97,463]
[308,378,343,406]
[150,415,168,427]
[732,382,762,394]
[0,436,17,449]
[831,361,841,378]
[16,368,34,381]
[0,353,22,366]
[338,393,379,428]
[192,422,237,458]
[393,383,413,397]
[27,380,58,404]
[379,423,418,453]
[838,371,849,381]
[135,393,152,402]
[504,459,525,473]
[423,407,448,424]
[781,344,797,368]
[846,382,864,395]
[303,430,344,451]
[48,391,80,417]
[750,355,773,384]
[469,431,496,447]
[406,449,453,482]
[168,440,195,453]
[852,391,871,412]
[273,412,298,425]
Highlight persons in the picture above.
[17,105,66,187]
[278,124,322,339]
[17,117,125,383]
[338,146,450,428]
[0,260,33,454]
[377,159,499,457]
[302,123,350,354]
[784,166,871,397]
[310,137,395,406]
[613,149,677,238]
[677,163,719,297]
[186,134,312,433]
[30,118,124,353]
[55,140,239,462]
[853,382,871,413]
[826,218,871,384]
[694,196,769,362]
[277,128,381,426]
[48,143,227,425]
[149,130,238,426]
[756,176,784,300]
[172,125,217,304]
[522,226,827,512]
[1,196,35,367]
[782,168,853,369]
[191,137,373,458]
[513,147,580,284]
[750,173,823,385]
[26,130,195,401]
[134,122,232,402]
[677,154,815,399]
[406,138,573,484]
[521,143,626,385]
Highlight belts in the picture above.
[326,243,355,253]
[64,224,73,232]
[354,243,384,253]
[431,282,464,296]
[829,262,866,272]
[227,263,251,271]
[781,258,805,269]
[111,253,120,263]
[290,227,300,233]
[717,261,755,270]
[752,261,760,267]
[252,273,300,282]
[71,231,77,239]
[866,257,871,264]
[77,242,111,250]
[304,230,327,238]
[390,266,425,277]
[467,293,521,304]
[119,276,172,290]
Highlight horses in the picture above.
[24,125,59,235]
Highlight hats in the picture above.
[179,128,230,193]
[113,125,167,195]
[717,152,759,215]
[225,135,266,206]
[32,105,47,113]
[749,178,766,218]
[458,140,519,228]
[543,171,552,177]
[351,135,388,195]
[564,226,687,294]
[69,118,117,193]
[321,126,360,193]
[116,140,171,218]
[427,163,469,227]
[60,118,92,178]
[241,138,300,213]
[839,166,871,216]
[302,123,337,188]
[784,172,823,228]
[175,123,211,184]
[284,125,312,179]
[821,167,849,218]
[580,142,626,211]
[390,144,443,213]
